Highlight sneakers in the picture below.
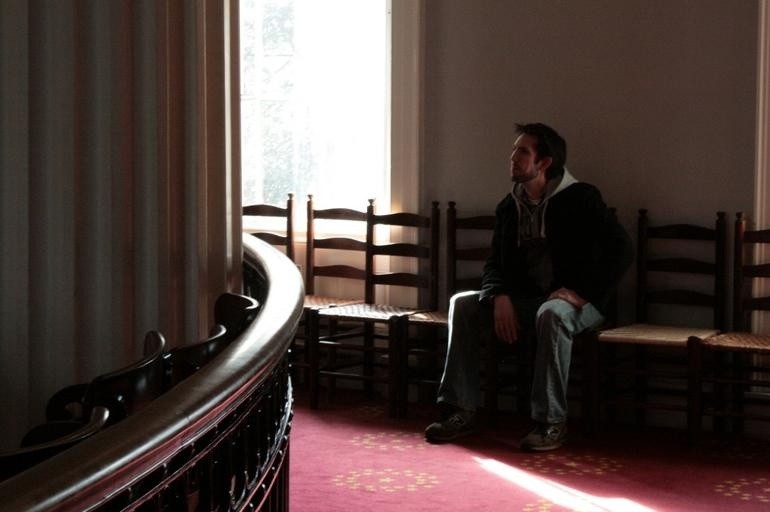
[423,409,483,443]
[523,420,569,452]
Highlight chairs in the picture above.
[705,210,768,445]
[290,194,371,392]
[18,328,168,451]
[1,405,119,482]
[397,199,495,419]
[307,199,443,418]
[237,194,299,266]
[213,292,262,348]
[591,208,732,445]
[491,205,618,435]
[170,323,229,389]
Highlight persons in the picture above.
[425,123,634,453]
